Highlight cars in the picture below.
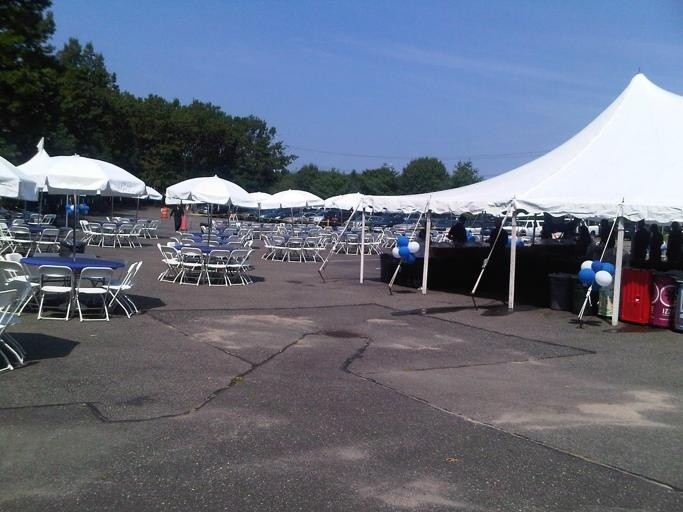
[0,203,40,230]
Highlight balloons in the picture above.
[577,260,615,290]
[392,236,420,264]
[466,230,475,242]
[65,203,90,215]
[507,235,524,249]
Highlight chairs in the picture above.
[0,251,145,322]
[1,279,39,376]
[0,203,595,288]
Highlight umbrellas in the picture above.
[249,192,272,223]
[261,188,325,237]
[0,156,38,202]
[131,185,163,217]
[22,153,147,263]
[15,137,50,221]
[165,197,210,224]
[324,192,371,227]
[166,174,258,224]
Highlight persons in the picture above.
[419,221,432,243]
[575,225,593,257]
[649,224,663,265]
[448,217,466,244]
[331,214,338,230]
[666,221,683,267]
[629,219,650,267]
[490,221,508,244]
[169,206,184,231]
[599,219,615,249]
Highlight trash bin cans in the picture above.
[160,207,169,217]
